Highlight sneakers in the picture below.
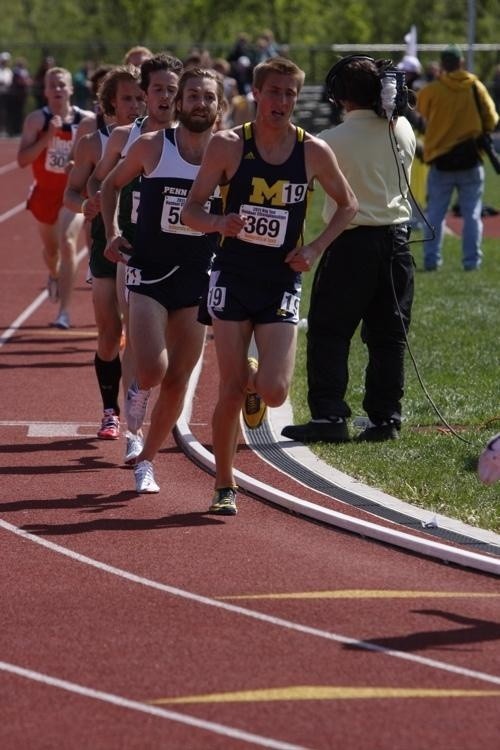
[280,415,349,442]
[209,483,238,515]
[123,380,152,463]
[97,408,120,440]
[242,357,267,430]
[354,421,399,442]
[48,275,70,329]
[134,459,160,493]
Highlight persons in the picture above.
[417,43,500,272]
[280,56,417,443]
[0,29,359,515]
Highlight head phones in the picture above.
[325,53,380,110]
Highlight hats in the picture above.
[441,42,464,62]
[398,56,422,74]
